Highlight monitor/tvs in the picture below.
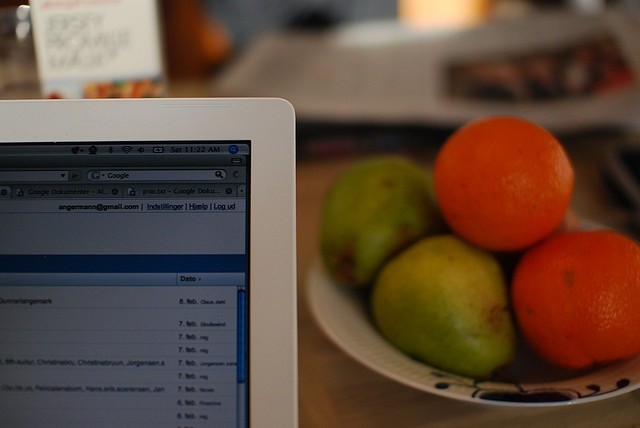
[0,97,298,428]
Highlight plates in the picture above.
[306,211,640,408]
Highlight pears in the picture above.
[319,155,438,291]
[369,233,521,386]
